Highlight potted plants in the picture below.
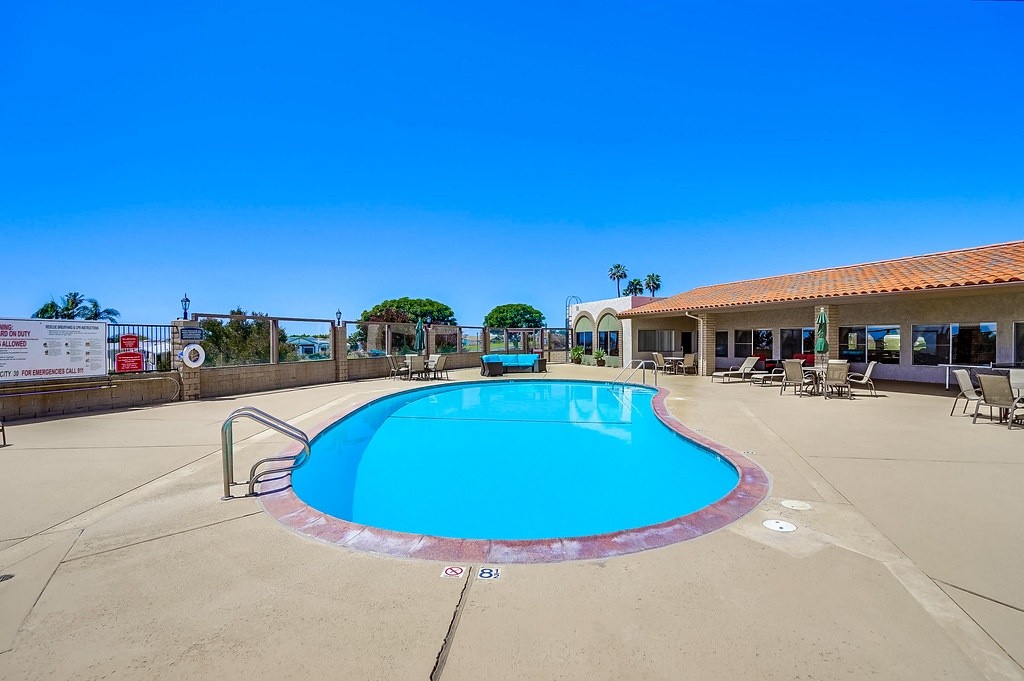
[569,345,584,363]
[593,347,605,366]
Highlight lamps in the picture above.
[545,323,547,328]
[336,308,342,326]
[181,293,190,320]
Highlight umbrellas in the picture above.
[413,318,426,356]
[814,307,830,371]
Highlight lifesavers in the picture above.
[183,343,206,369]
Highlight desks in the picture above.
[483,362,504,377]
[664,357,684,375]
[404,360,434,380]
[802,366,827,396]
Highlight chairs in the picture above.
[712,357,877,400]
[652,352,698,376]
[386,353,449,381]
[954,368,1024,429]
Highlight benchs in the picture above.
[479,354,547,373]
